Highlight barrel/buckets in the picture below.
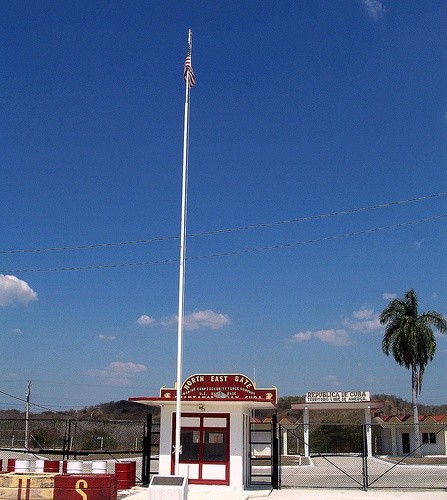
[43,460,59,472]
[7,458,15,471]
[14,460,31,472]
[35,460,44,472]
[59,459,137,490]
[0,459,3,471]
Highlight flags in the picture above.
[183,29,197,88]
